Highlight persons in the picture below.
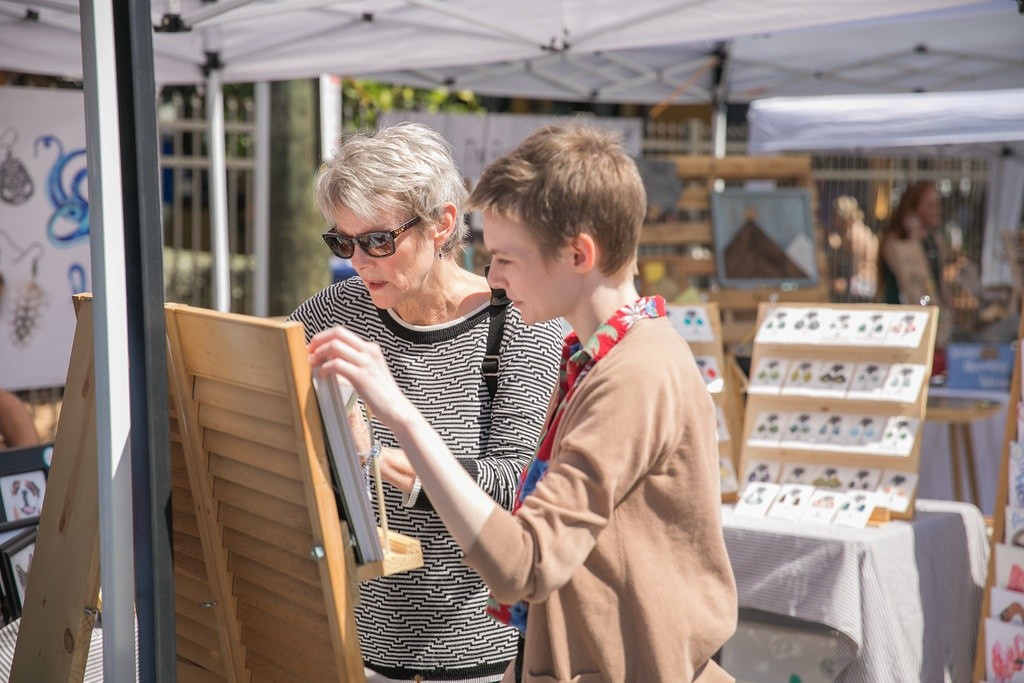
[829,194,878,301]
[0,388,41,451]
[307,125,736,683]
[287,120,562,682]
[879,181,948,347]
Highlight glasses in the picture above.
[322,217,421,259]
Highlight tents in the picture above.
[745,88,1023,289]
[1,0,1023,683]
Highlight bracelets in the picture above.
[362,444,381,501]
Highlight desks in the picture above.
[711,489,988,683]
